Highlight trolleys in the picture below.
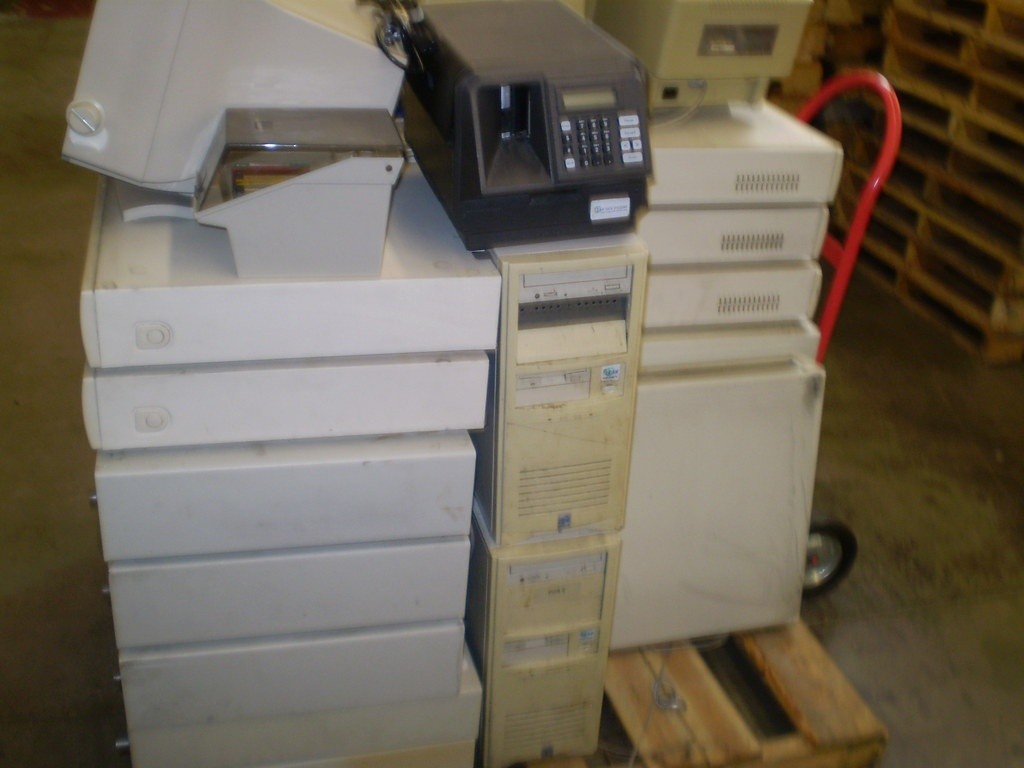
[790,67,904,598]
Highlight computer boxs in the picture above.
[471,230,649,546]
[471,495,622,768]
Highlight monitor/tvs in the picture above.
[581,0,812,113]
[62,0,409,198]
